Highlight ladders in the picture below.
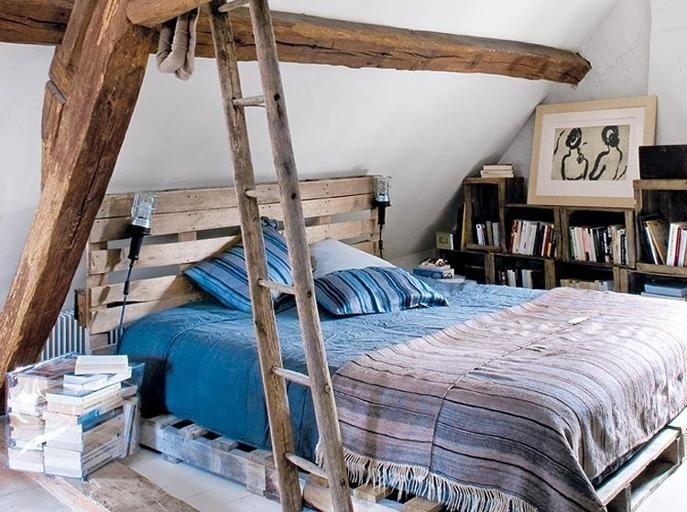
[208,0,352,512]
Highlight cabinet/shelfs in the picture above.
[432,177,687,301]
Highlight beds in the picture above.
[72,173,687,512]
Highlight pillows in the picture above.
[181,213,314,313]
[312,267,450,318]
[305,235,398,280]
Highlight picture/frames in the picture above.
[524,93,659,211]
[433,229,454,251]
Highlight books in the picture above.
[4,351,147,479]
[474,164,687,301]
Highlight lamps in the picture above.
[115,192,159,349]
[369,175,392,260]
